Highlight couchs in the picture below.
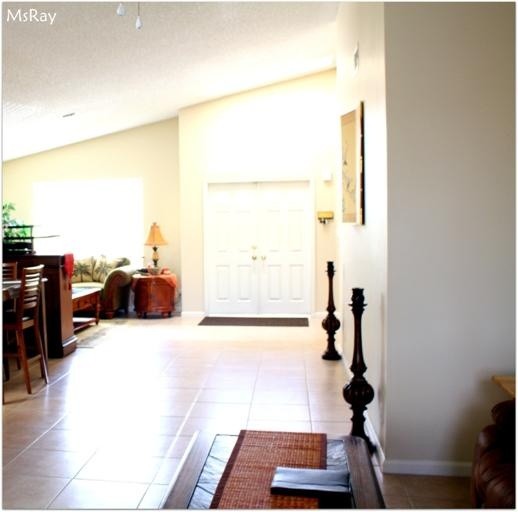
[70,255,137,321]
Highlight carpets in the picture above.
[197,315,309,328]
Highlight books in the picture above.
[268,467,351,498]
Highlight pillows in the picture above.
[71,258,94,283]
[93,258,126,284]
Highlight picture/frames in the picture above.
[338,99,366,225]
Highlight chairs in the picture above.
[464,399,515,508]
[2,262,50,395]
[2,261,22,369]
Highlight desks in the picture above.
[72,288,104,333]
[2,277,53,378]
[489,373,515,399]
[157,428,386,508]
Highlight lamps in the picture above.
[316,210,334,225]
[116,4,145,31]
[144,223,169,273]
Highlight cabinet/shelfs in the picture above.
[131,274,177,318]
